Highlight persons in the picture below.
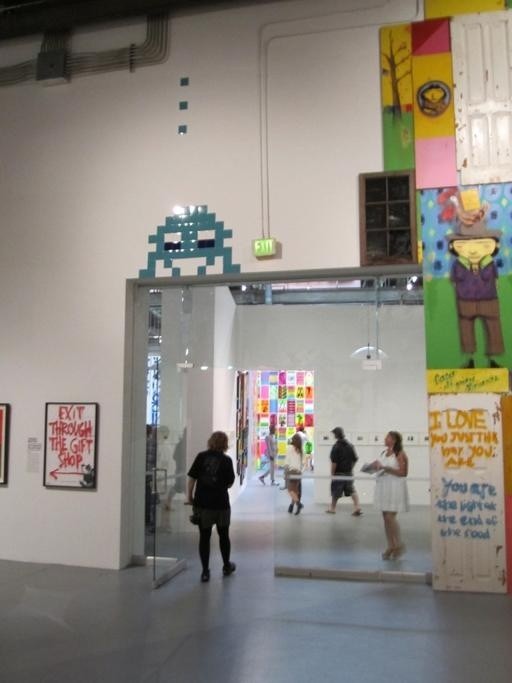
[304,440,315,471]
[374,428,410,559]
[284,432,305,514]
[258,423,279,487]
[447,214,507,367]
[326,426,363,513]
[146,421,170,532]
[183,430,236,582]
[163,427,188,510]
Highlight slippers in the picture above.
[325,508,366,515]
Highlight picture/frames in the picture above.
[0,403,10,484]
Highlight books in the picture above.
[360,461,383,474]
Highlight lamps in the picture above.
[350,280,387,360]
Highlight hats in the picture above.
[445,217,501,241]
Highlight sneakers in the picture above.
[381,543,408,561]
[288,501,304,516]
[201,561,236,582]
